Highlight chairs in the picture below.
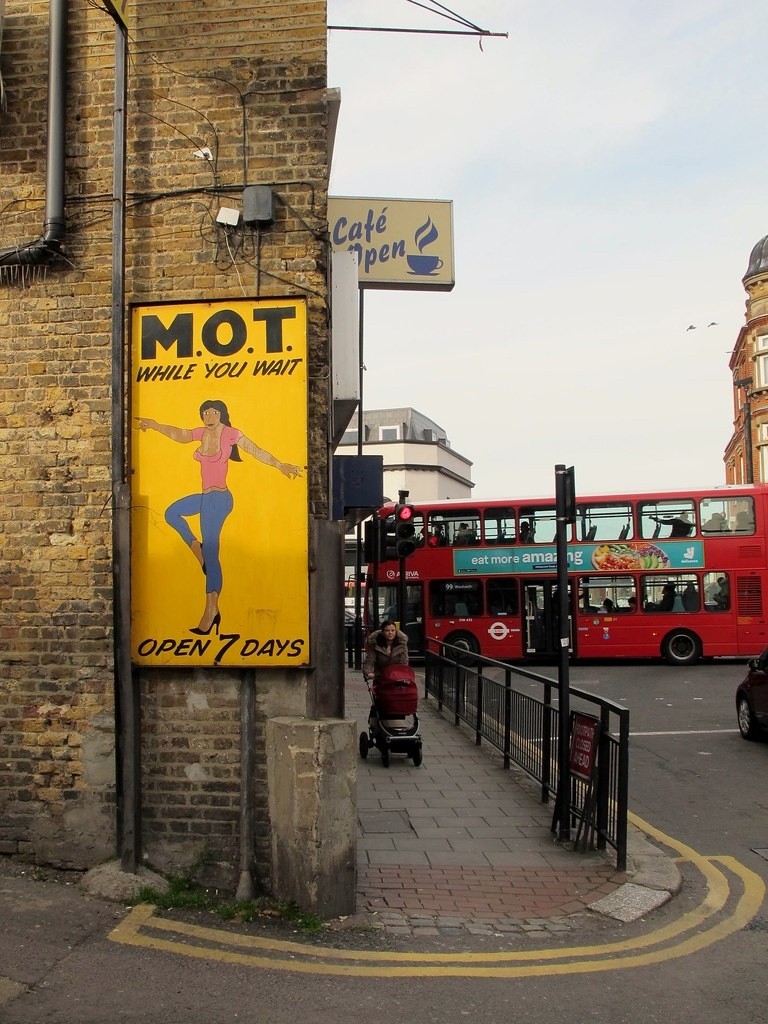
[462,531,479,545]
[495,530,506,545]
[618,524,631,541]
[673,595,687,612]
[524,528,536,545]
[587,524,597,540]
[651,523,663,538]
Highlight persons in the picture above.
[569,591,586,613]
[734,512,749,532]
[520,522,531,541]
[650,511,695,536]
[362,620,408,728]
[713,578,729,606]
[682,582,699,609]
[433,524,445,544]
[603,599,612,613]
[702,513,727,531]
[420,527,428,544]
[456,523,471,546]
[654,583,676,611]
[628,597,638,612]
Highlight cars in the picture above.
[735,646,768,742]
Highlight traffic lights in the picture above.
[395,501,416,558]
[379,517,396,561]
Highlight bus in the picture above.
[344,534,387,627]
[363,481,768,669]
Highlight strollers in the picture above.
[358,663,423,768]
